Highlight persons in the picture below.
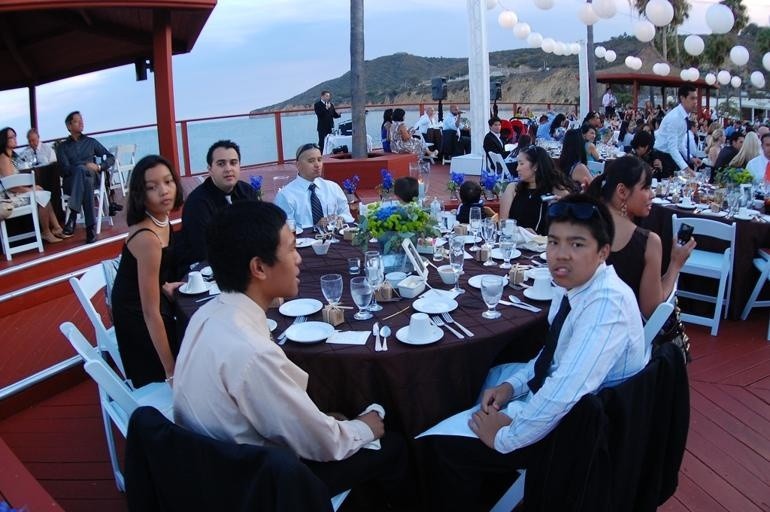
[182,140,258,254]
[651,86,702,178]
[405,194,646,512]
[710,131,744,187]
[441,103,472,155]
[630,131,682,180]
[418,108,436,140]
[314,91,342,155]
[389,108,438,164]
[0,127,74,244]
[273,143,350,230]
[56,112,115,244]
[19,129,57,167]
[548,113,567,142]
[584,156,697,321]
[110,155,183,389]
[501,146,571,237]
[679,108,770,157]
[579,124,600,163]
[705,128,725,166]
[494,85,671,138]
[381,109,393,152]
[455,181,495,224]
[483,117,518,179]
[728,132,770,194]
[394,176,419,209]
[556,129,593,195]
[608,119,634,148]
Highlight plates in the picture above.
[396,324,444,345]
[456,235,482,244]
[178,282,212,294]
[468,274,508,289]
[296,238,315,247]
[284,321,334,343]
[523,288,556,299]
[278,298,323,316]
[527,268,552,280]
[491,248,521,259]
[540,252,547,260]
[677,203,695,209]
[412,298,458,314]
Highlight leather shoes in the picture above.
[62,215,77,238]
[86,227,96,242]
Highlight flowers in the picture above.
[343,176,359,193]
[354,206,440,252]
[714,167,754,187]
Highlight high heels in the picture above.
[429,149,438,158]
[52,227,73,238]
[42,232,63,243]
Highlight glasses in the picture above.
[527,144,536,151]
[296,143,321,161]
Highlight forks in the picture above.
[509,283,529,291]
[276,314,307,346]
[442,312,474,337]
[432,316,464,339]
[522,255,540,259]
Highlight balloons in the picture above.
[576,2,599,26]
[634,22,656,43]
[717,71,731,86]
[483,0,583,59]
[705,73,716,86]
[730,45,749,67]
[604,50,617,64]
[594,46,606,60]
[684,34,705,57]
[592,0,618,21]
[750,71,765,88]
[624,56,642,71]
[680,67,700,82]
[730,75,742,89]
[705,4,734,34]
[646,0,674,27]
[762,52,770,72]
[652,63,670,78]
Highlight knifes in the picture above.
[498,300,538,313]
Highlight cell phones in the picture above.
[677,222,694,246]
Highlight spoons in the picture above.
[509,295,541,312]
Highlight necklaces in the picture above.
[143,210,170,229]
[527,187,538,199]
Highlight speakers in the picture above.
[430,76,449,102]
[490,81,502,100]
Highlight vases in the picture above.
[377,232,416,273]
[346,193,355,204]
[725,183,736,197]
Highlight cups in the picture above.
[481,276,504,320]
[437,264,463,284]
[483,217,497,267]
[350,276,374,320]
[410,162,430,194]
[481,223,494,244]
[365,251,384,312]
[499,236,516,269]
[500,219,517,237]
[682,197,690,206]
[448,236,465,294]
[187,271,205,291]
[348,258,361,276]
[468,207,481,252]
[433,245,445,262]
[533,276,551,295]
[384,272,407,289]
[407,313,431,341]
[312,240,331,256]
[326,203,340,243]
[320,274,343,306]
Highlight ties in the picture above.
[308,185,324,224]
[685,117,690,162]
[526,295,571,395]
[225,195,232,205]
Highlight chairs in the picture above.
[59,322,177,491]
[671,214,737,337]
[1,170,45,262]
[697,130,708,152]
[110,145,135,197]
[740,247,770,343]
[411,130,436,165]
[65,155,114,234]
[642,271,681,347]
[586,160,606,177]
[489,151,512,182]
[70,255,126,381]
[440,128,466,166]
